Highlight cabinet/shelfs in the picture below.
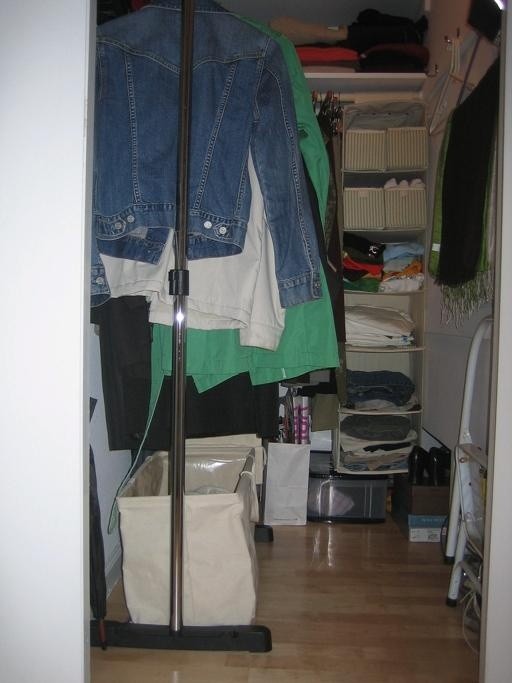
[305,72,432,475]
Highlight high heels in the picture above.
[407,446,428,484]
[427,446,443,487]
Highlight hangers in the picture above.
[429,37,478,135]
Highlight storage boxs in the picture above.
[311,448,388,525]
